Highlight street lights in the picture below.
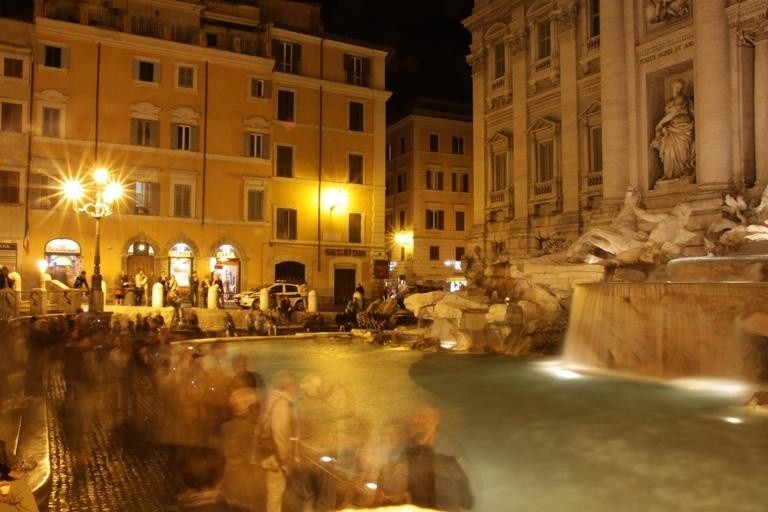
[394,225,409,286]
[63,167,123,274]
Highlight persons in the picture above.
[0,266,17,317]
[73,271,89,291]
[211,272,225,310]
[201,275,211,307]
[564,185,691,271]
[166,273,182,308]
[372,405,473,512]
[157,270,169,308]
[652,76,694,180]
[188,271,199,307]
[0,478,40,512]
[113,269,129,306]
[134,268,148,306]
[0,440,38,481]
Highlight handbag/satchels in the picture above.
[257,438,276,459]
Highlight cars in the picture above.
[233,279,305,312]
